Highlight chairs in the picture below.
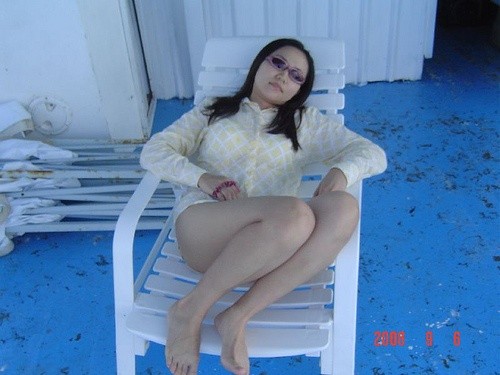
[111,37,363,375]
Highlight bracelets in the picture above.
[212,180,235,198]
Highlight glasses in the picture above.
[265,56,305,85]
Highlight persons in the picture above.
[140,39,388,375]
